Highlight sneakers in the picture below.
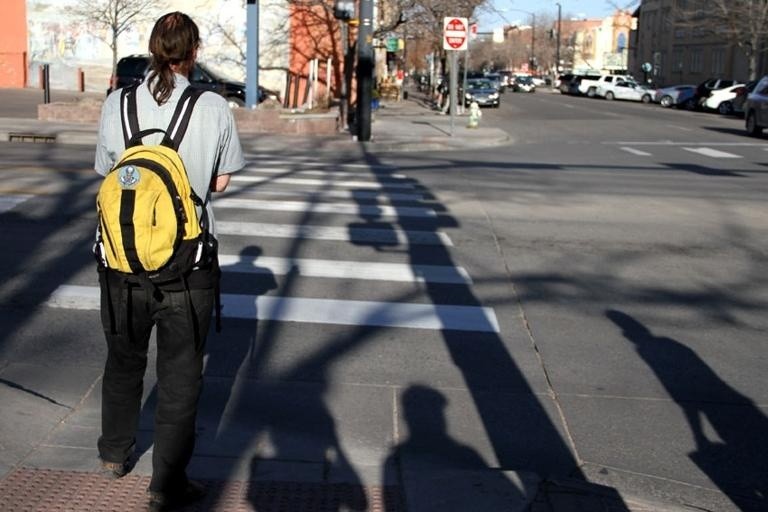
[150,481,205,512]
[103,461,130,477]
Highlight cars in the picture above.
[460,77,500,107]
[656,82,699,108]
[494,72,547,93]
[698,75,768,133]
[558,73,656,103]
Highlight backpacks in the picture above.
[94,83,217,291]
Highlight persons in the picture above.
[343,39,360,141]
[97,11,245,511]
[419,71,428,92]
[438,79,448,98]
[395,67,404,86]
